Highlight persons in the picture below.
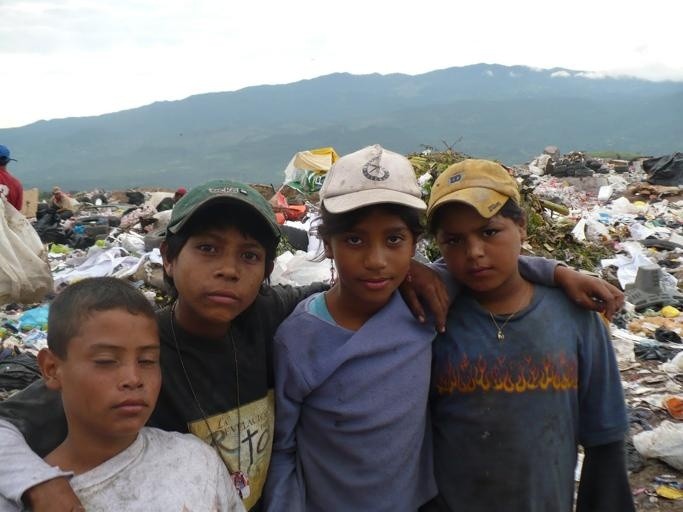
[155,186,187,212]
[49,184,80,219]
[427,156,638,512]
[261,142,627,512]
[0,276,249,512]
[0,174,452,512]
[0,143,24,212]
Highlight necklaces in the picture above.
[489,283,532,341]
[168,296,250,500]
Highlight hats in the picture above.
[320,143,427,215]
[167,179,283,238]
[52,186,62,194]
[426,158,521,233]
[0,144,11,157]
